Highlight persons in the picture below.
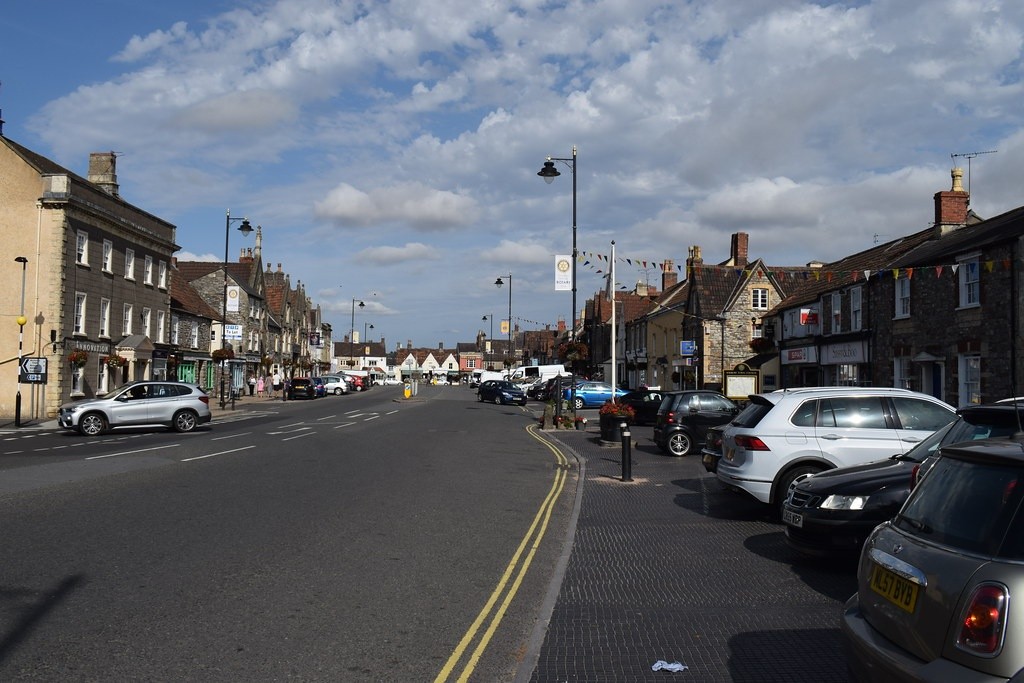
[271,368,283,399]
[265,372,273,398]
[257,376,265,398]
[248,373,256,396]
[425,373,433,388]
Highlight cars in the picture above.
[519,372,591,401]
[384,378,404,387]
[617,389,667,426]
[654,390,744,458]
[313,370,384,399]
[429,379,450,386]
[288,378,318,400]
[561,382,632,410]
[701,407,872,475]
[477,379,528,406]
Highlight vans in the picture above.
[503,365,566,383]
[469,369,518,388]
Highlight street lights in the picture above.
[364,322,374,366]
[481,313,493,370]
[220,207,254,409]
[14,256,29,425]
[494,271,512,381]
[537,144,579,413]
[350,299,365,369]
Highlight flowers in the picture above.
[104,354,126,369]
[212,349,234,363]
[599,393,635,421]
[64,350,88,369]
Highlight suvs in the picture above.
[837,402,1024,682]
[782,398,1023,560]
[57,381,212,438]
[717,386,991,521]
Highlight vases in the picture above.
[599,413,629,441]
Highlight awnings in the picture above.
[449,370,459,377]
[369,370,381,375]
[401,370,423,376]
[432,370,448,377]
[460,370,472,377]
